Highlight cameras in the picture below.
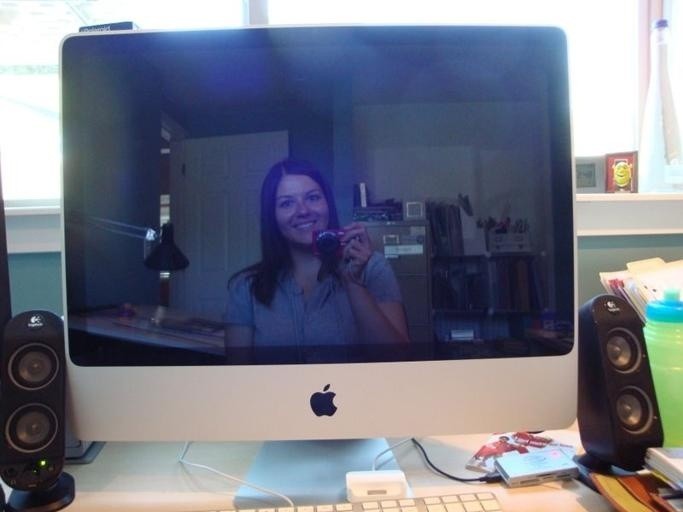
[311,228,349,257]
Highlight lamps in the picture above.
[86,214,189,271]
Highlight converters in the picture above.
[494,447,579,488]
[346,469,408,501]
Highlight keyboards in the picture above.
[208,491,502,512]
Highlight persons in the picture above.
[221,157,411,364]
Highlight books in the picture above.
[640,444,683,495]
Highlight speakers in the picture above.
[0,309,75,511]
[574,294,665,475]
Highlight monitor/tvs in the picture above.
[56,24,584,506]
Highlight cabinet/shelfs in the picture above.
[432,250,547,342]
[366,219,434,344]
